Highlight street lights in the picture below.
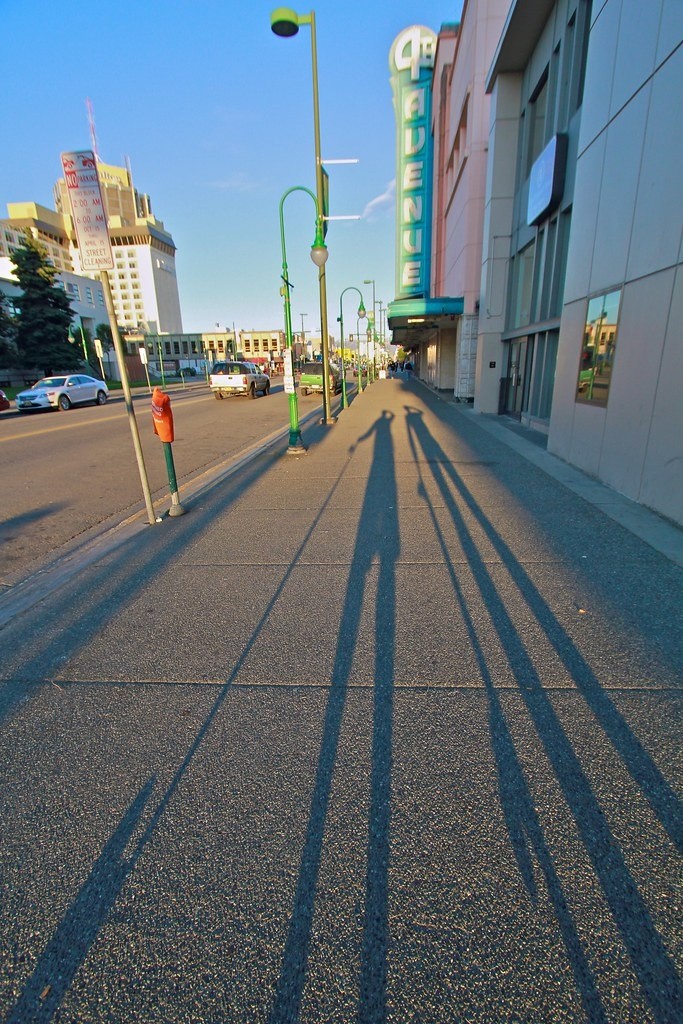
[277,185,330,455]
[363,279,377,382]
[194,338,210,385]
[146,330,166,391]
[337,285,366,410]
[379,308,386,371]
[374,299,383,370]
[67,323,92,377]
[356,316,372,395]
[269,4,338,424]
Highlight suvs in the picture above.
[208,360,272,401]
[299,361,346,397]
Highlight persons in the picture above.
[388,361,413,381]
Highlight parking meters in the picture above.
[180,370,186,388]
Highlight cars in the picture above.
[353,364,368,377]
[14,373,110,413]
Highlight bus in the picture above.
[579,342,598,393]
